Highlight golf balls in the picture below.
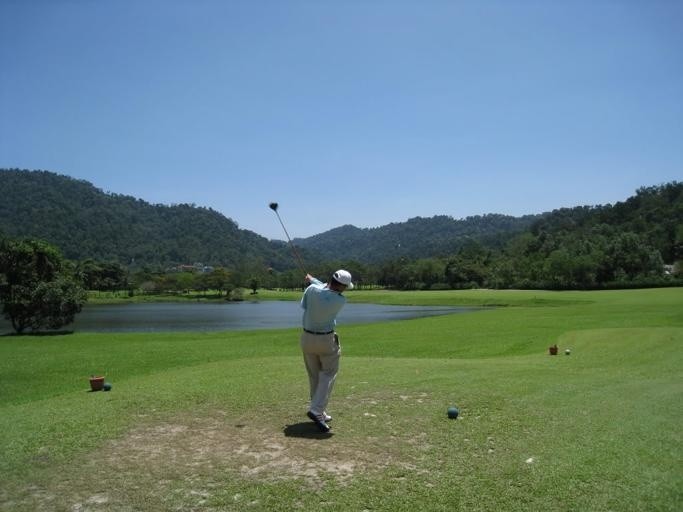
[565,349,571,355]
[104,383,111,389]
[448,408,458,419]
[270,203,307,276]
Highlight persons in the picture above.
[299,269,354,432]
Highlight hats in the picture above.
[332,270,354,290]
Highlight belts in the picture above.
[303,328,333,334]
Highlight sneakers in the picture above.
[322,411,331,421]
[306,409,329,431]
